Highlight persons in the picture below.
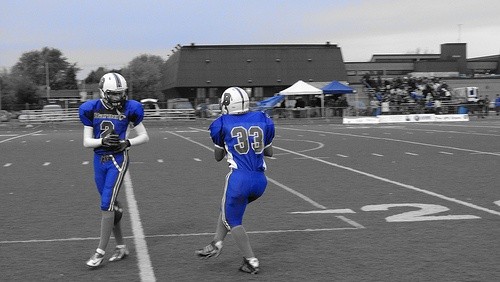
[327,97,349,115]
[295,98,305,108]
[193,85,275,275]
[280,99,286,108]
[76,71,150,268]
[359,73,500,120]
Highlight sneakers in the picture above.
[196,240,223,258]
[239,256,260,275]
[108,245,129,261]
[86,248,106,267]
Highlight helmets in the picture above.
[97,72,128,112]
[219,87,250,115]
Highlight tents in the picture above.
[320,80,359,117]
[276,79,325,119]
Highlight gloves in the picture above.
[110,138,127,154]
[102,133,119,146]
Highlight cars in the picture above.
[139,98,160,121]
[18,111,37,123]
[41,105,63,123]
[195,102,222,118]
[165,102,196,120]
